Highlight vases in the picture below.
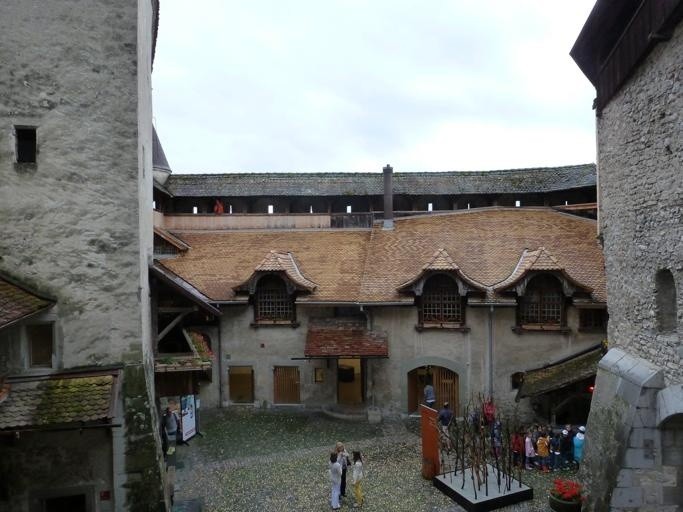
[549,495,582,512]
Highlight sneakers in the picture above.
[339,496,342,500]
[340,493,347,497]
[524,464,574,473]
[331,503,341,510]
[353,503,361,507]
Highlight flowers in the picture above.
[551,477,582,501]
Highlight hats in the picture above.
[561,430,568,435]
[578,426,586,433]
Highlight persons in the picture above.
[327,452,344,509]
[351,449,364,507]
[166,397,179,412]
[463,395,502,462]
[333,442,351,499]
[422,382,436,408]
[435,402,454,450]
[158,410,175,455]
[212,198,224,214]
[509,422,586,473]
[161,407,180,448]
